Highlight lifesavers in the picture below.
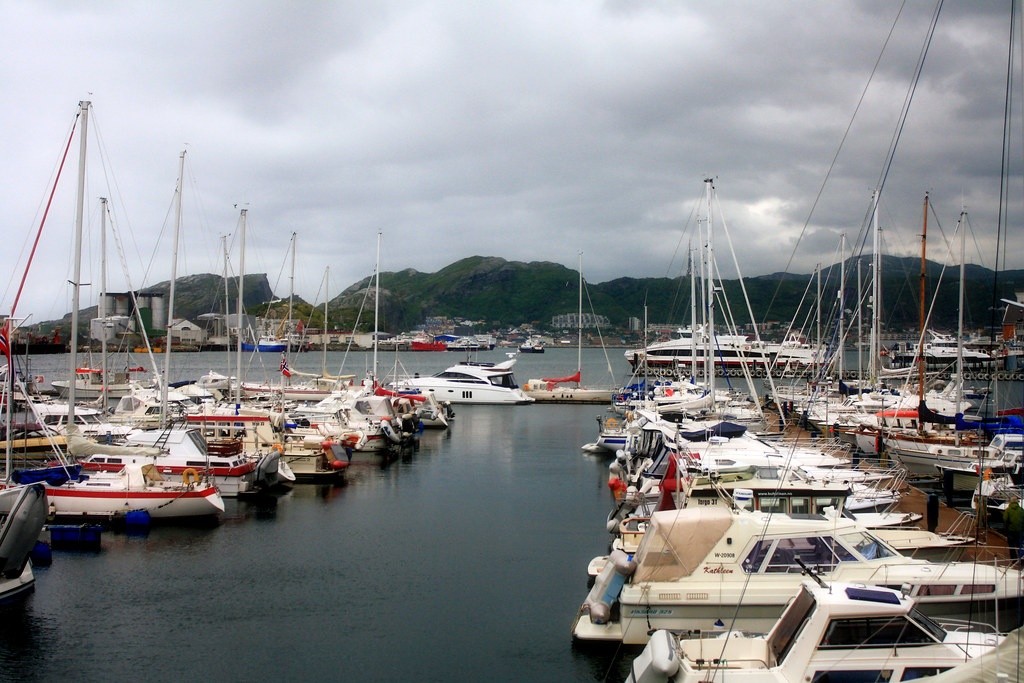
[270,444,282,453]
[182,468,199,484]
[607,418,617,428]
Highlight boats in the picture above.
[517,332,546,353]
[386,327,537,406]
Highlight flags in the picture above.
[279,354,291,378]
[0,320,14,381]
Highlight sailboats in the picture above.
[520,249,623,403]
[0,87,497,601]
[570,1,1024,683]
